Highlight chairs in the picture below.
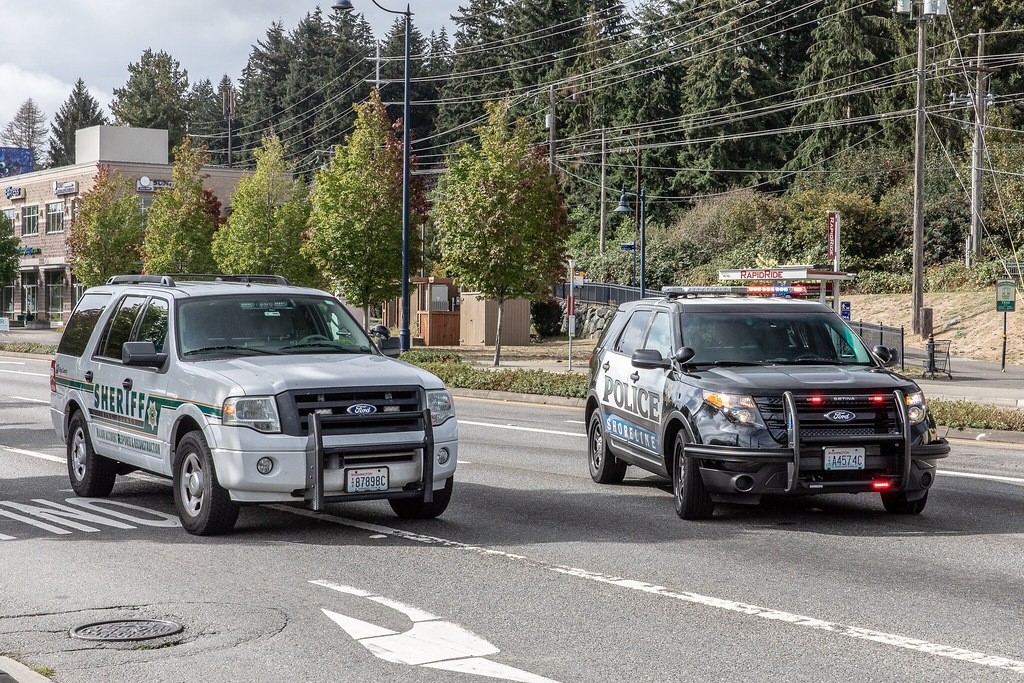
[756,328,791,362]
[257,315,294,347]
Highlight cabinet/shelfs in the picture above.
[921,340,953,381]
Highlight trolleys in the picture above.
[920,338,953,382]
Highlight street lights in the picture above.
[566,260,579,371]
[619,241,639,299]
[330,0,416,353]
[614,181,647,299]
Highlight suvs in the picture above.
[583,286,953,522]
[47,273,459,538]
